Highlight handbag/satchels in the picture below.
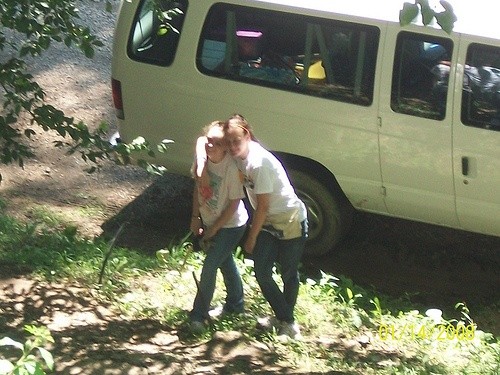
[266,208,302,240]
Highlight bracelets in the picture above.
[191,216,201,220]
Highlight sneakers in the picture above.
[209,309,245,317]
[257,315,281,330]
[281,318,300,340]
[192,320,208,327]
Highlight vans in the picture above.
[111,0,500,256]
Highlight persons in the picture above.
[195,114,308,342]
[180,120,249,331]
